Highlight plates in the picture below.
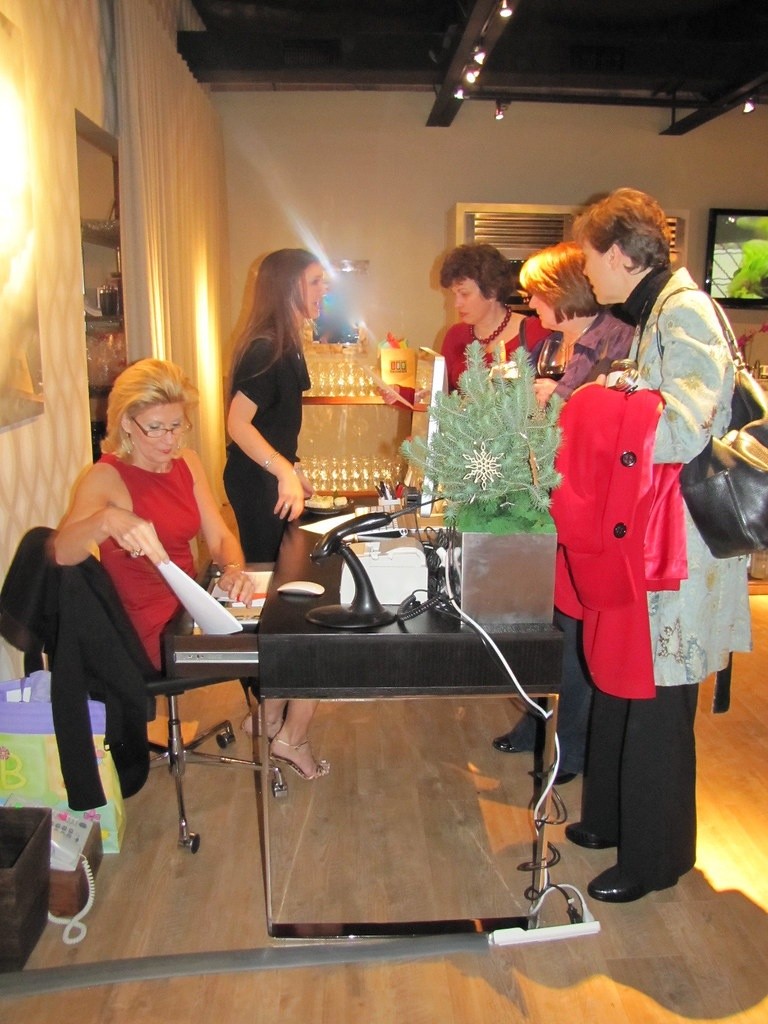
[305,499,357,514]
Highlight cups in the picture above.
[537,338,569,381]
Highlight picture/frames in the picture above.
[704,207,768,310]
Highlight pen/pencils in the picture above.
[375,481,397,500]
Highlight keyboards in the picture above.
[354,504,409,536]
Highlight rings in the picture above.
[131,548,142,558]
[284,503,289,508]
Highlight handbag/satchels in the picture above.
[657,288,768,559]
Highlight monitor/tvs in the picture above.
[412,345,448,517]
[704,208,768,310]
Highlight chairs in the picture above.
[11,525,289,856]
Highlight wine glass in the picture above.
[294,363,408,491]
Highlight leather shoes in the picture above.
[565,822,620,849]
[587,863,679,903]
[554,770,576,783]
[493,733,519,752]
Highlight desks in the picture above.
[158,501,562,937]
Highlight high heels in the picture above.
[239,676,253,709]
[251,678,288,721]
[239,713,288,746]
[268,735,332,781]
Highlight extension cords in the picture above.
[488,921,600,945]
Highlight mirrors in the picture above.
[74,110,128,464]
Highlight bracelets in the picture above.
[262,451,280,471]
[223,562,241,569]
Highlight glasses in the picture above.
[130,416,192,438]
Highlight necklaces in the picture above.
[559,322,594,363]
[635,300,649,335]
[470,304,512,343]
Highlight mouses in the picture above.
[277,581,325,595]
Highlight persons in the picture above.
[440,239,552,396]
[223,247,329,720]
[55,357,331,781]
[566,186,752,904]
[491,243,634,785]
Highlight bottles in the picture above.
[109,272,122,316]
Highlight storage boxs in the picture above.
[0,805,52,970]
